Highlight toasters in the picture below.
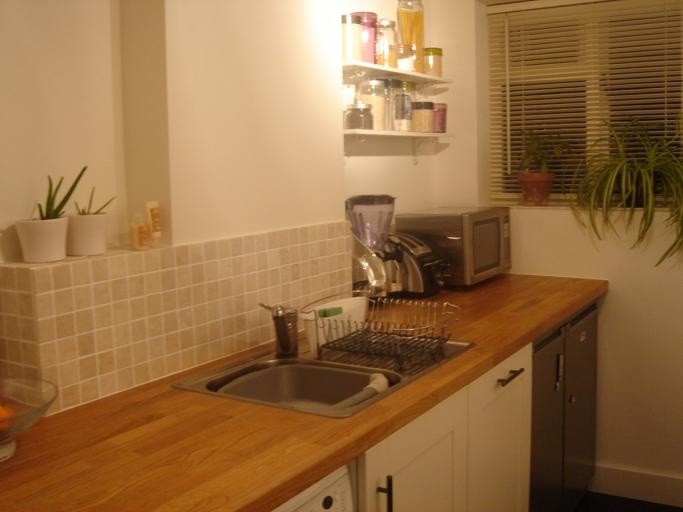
[387,231,452,296]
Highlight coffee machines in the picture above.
[344,194,408,297]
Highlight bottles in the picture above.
[397,1,425,74]
[374,19,397,67]
[424,48,442,77]
[345,78,446,133]
[129,206,162,251]
[341,13,378,65]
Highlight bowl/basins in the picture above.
[0,376,58,462]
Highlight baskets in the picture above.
[316,294,462,376]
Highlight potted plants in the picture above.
[570,112,683,270]
[64,185,119,257]
[514,121,568,208]
[10,163,89,265]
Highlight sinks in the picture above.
[311,326,476,379]
[172,359,408,420]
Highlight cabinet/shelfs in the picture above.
[268,457,355,510]
[467,341,533,512]
[343,60,454,158]
[349,383,468,512]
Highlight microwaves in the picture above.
[391,205,513,287]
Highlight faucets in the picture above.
[258,302,299,361]
